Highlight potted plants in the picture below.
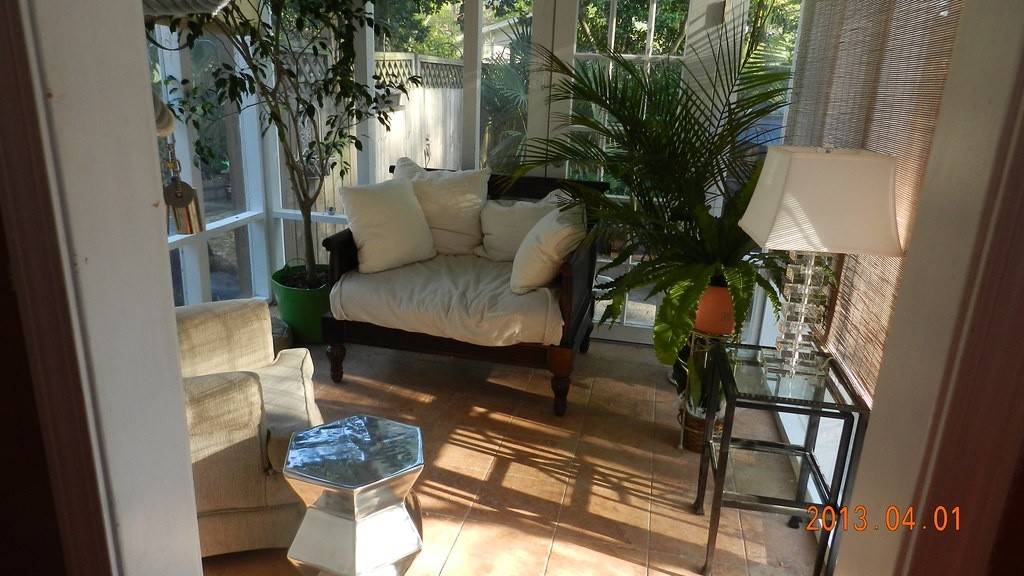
[146,1,424,345]
[493,0,839,452]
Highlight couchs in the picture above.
[175,299,325,558]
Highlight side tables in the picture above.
[282,412,424,576]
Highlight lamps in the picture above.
[738,146,903,376]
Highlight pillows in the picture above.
[475,189,573,262]
[339,177,437,274]
[393,158,491,255]
[510,199,588,294]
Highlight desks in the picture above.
[694,343,871,576]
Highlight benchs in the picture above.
[321,165,610,417]
